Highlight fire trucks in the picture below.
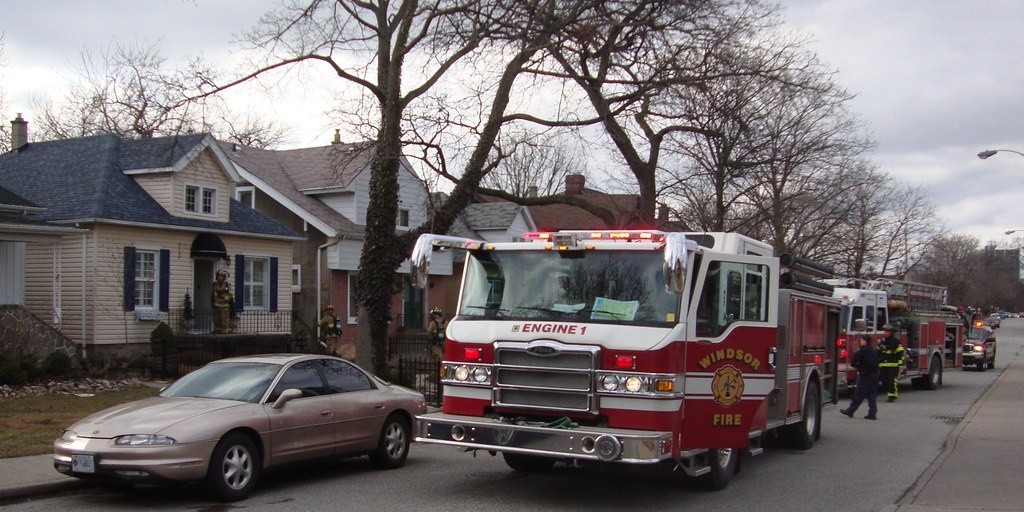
[411,228,842,491]
[818,278,962,390]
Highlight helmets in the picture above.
[882,324,897,331]
[325,304,335,311]
[430,306,441,315]
[216,270,228,280]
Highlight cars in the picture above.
[963,310,1024,372]
[54,354,426,500]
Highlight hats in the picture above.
[862,334,872,343]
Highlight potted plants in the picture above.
[229,298,243,333]
[182,287,196,334]
[150,321,178,377]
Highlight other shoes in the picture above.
[840,409,853,417]
[887,396,895,402]
[865,414,876,420]
[210,329,223,334]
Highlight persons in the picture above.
[877,324,905,402]
[840,334,879,421]
[209,269,236,335]
[317,304,338,356]
[427,306,449,361]
